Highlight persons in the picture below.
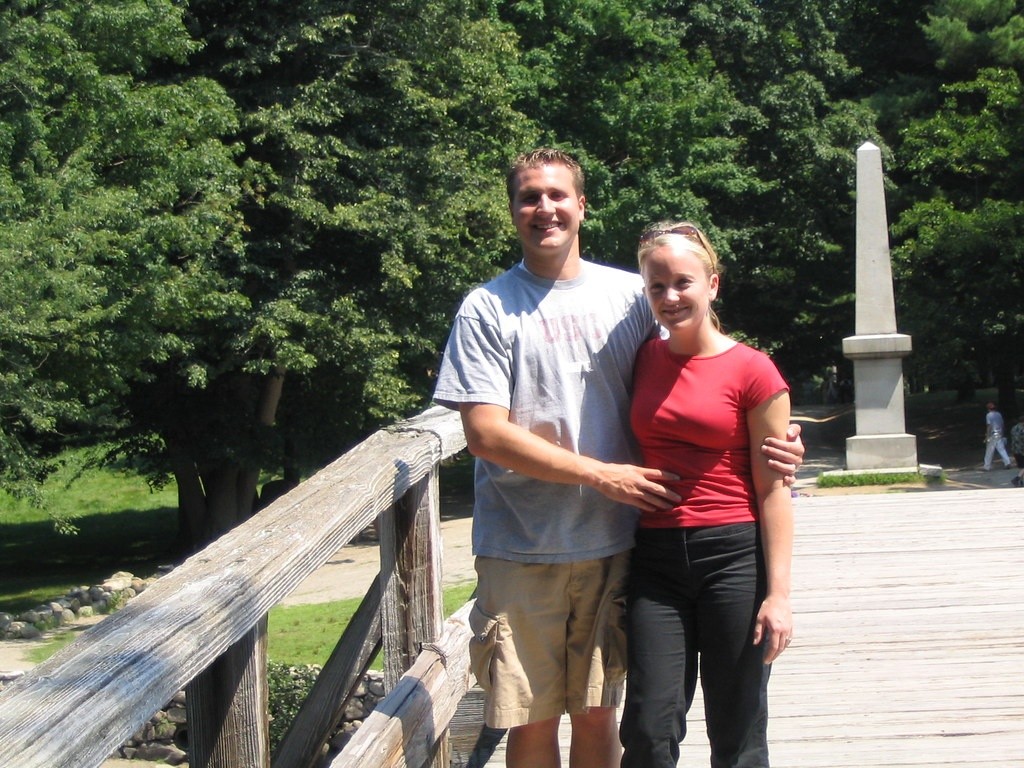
[1010,416,1024,488]
[618,219,793,768]
[979,401,1011,471]
[821,359,843,404]
[433,149,804,768]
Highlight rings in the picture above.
[786,637,792,642]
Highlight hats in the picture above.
[988,403,995,410]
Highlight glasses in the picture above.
[641,225,714,275]
